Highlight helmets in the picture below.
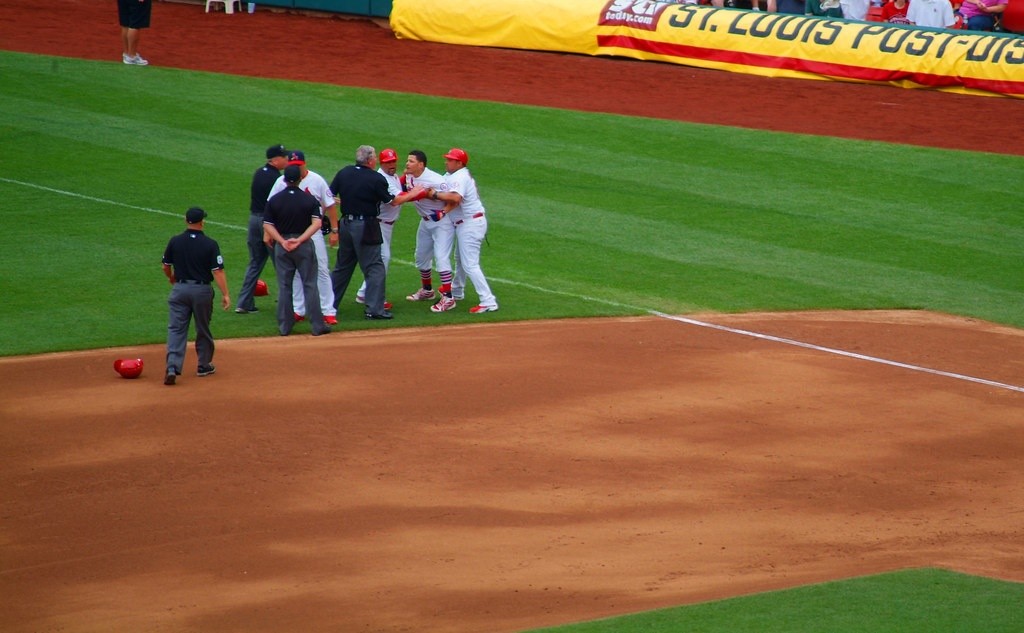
[379,149,397,163]
[253,279,268,296]
[114,359,144,379]
[444,149,468,164]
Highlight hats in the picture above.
[288,151,304,166]
[267,144,290,159]
[185,208,207,223]
[284,166,300,182]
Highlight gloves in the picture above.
[429,209,446,221]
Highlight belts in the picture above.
[341,214,376,220]
[175,279,209,284]
[376,218,394,224]
[422,216,433,221]
[251,211,262,216]
[455,213,483,224]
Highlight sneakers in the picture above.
[469,304,498,313]
[294,313,305,322]
[164,366,176,384]
[364,310,392,320]
[383,301,393,310]
[324,316,338,324]
[431,295,456,313]
[450,294,464,300]
[356,295,366,304]
[406,288,436,302]
[235,305,257,314]
[121,51,148,66]
[196,362,215,376]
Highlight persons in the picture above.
[162,206,231,385]
[235,145,499,336]
[118,1,150,66]
[651,0,1024,34]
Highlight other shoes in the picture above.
[319,326,330,334]
